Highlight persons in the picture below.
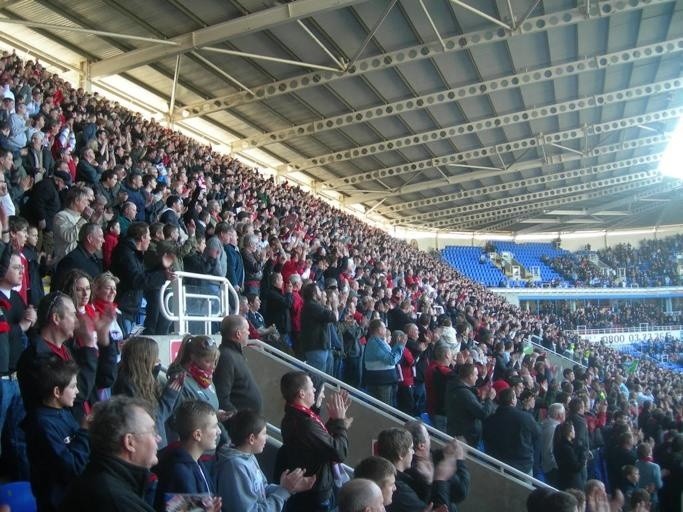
[0,48,682,511]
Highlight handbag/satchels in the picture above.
[364,361,404,385]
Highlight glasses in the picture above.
[201,337,216,350]
[48,290,65,315]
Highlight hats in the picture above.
[53,170,73,187]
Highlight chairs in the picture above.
[0,476,38,511]
[439,239,683,371]
